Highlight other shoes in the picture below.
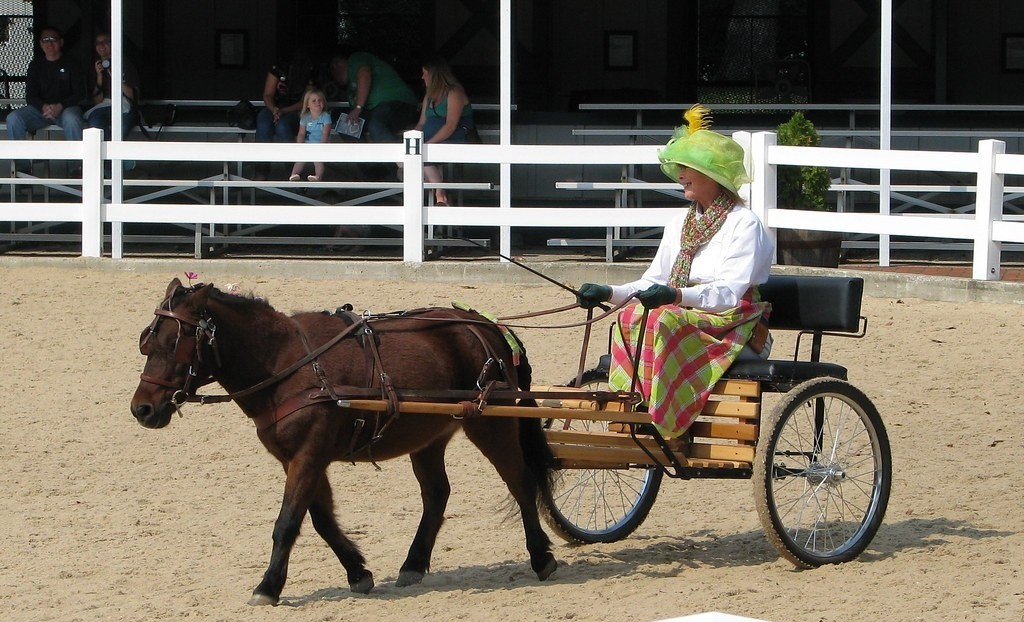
[308,175,320,181]
[290,175,300,181]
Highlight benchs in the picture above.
[547,106,1024,266]
[2,98,519,257]
[600,275,869,381]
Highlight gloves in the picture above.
[635,283,677,310]
[576,283,611,309]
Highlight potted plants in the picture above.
[773,111,844,269]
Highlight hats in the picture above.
[657,103,756,198]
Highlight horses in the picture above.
[130,278,565,606]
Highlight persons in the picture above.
[289,85,333,182]
[88,32,139,179]
[396,56,483,207]
[6,27,88,189]
[576,103,773,452]
[329,52,417,169]
[252,42,321,181]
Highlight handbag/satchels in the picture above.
[161,104,178,125]
[465,131,483,144]
[227,97,259,129]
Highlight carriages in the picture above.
[132,272,890,606]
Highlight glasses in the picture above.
[40,37,59,43]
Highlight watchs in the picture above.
[356,104,364,110]
